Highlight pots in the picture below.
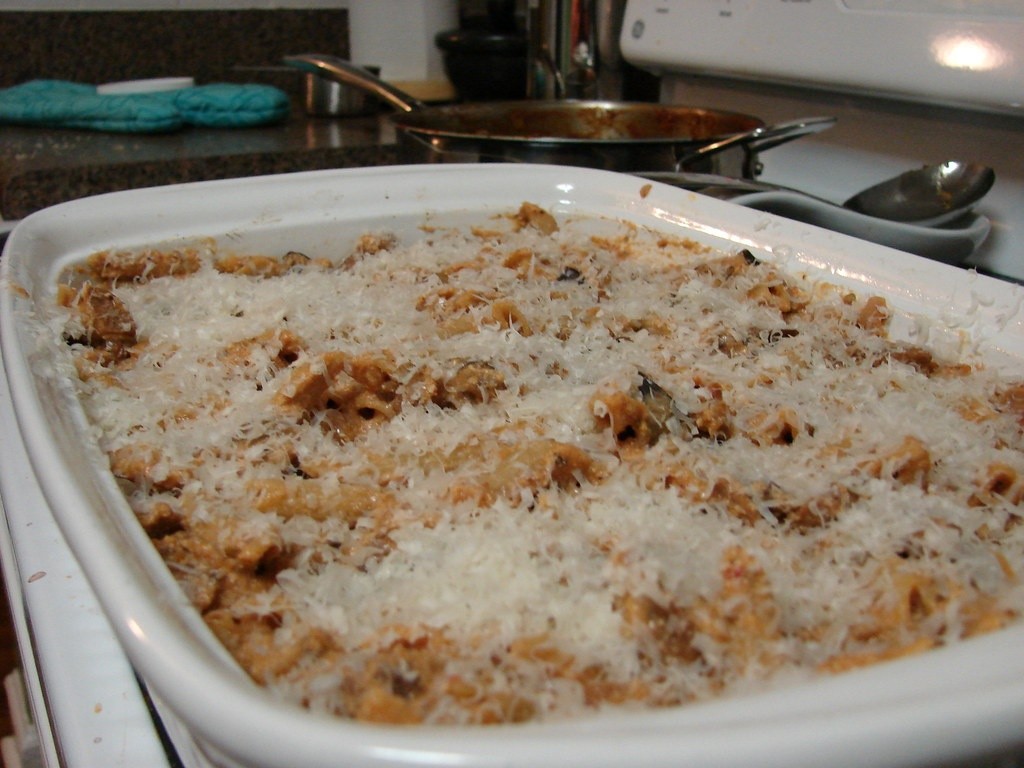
[285,50,838,181]
[226,64,381,116]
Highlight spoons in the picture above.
[626,160,997,265]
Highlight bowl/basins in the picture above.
[0,163,1024,768]
[435,16,526,102]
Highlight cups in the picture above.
[537,0,627,101]
